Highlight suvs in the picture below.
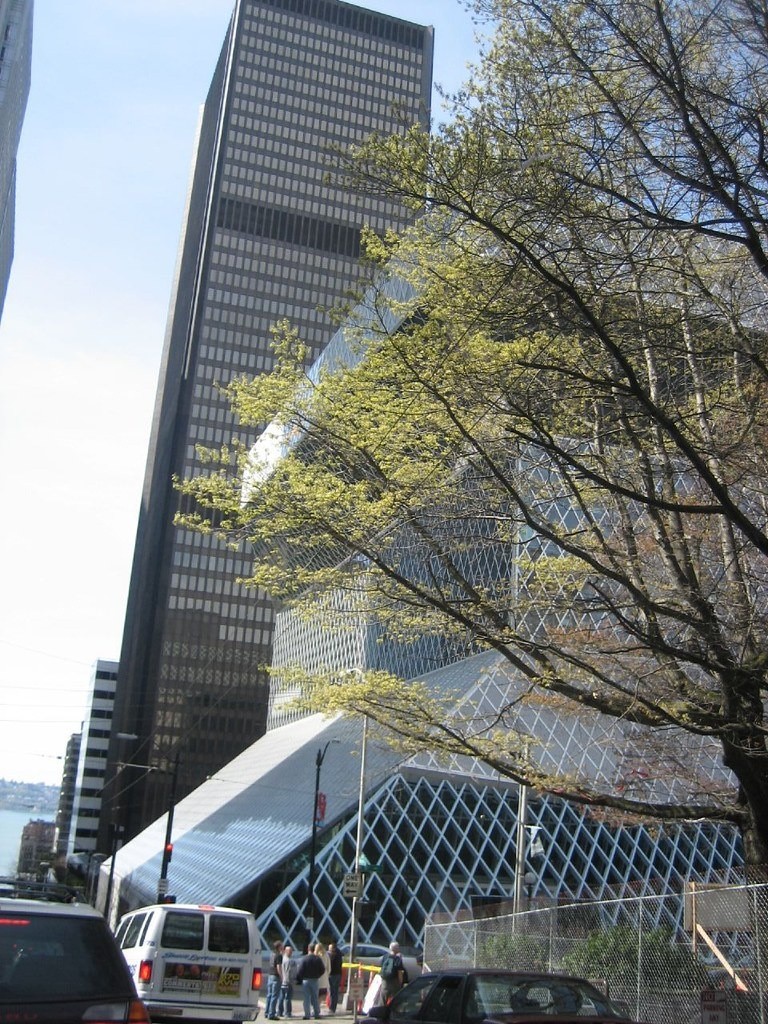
[0,876,154,1024]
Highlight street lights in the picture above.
[116,732,179,904]
[339,668,372,1011]
[525,872,537,913]
[303,737,340,956]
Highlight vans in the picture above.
[115,903,262,1024]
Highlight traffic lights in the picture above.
[166,844,172,862]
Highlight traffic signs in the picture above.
[344,873,364,898]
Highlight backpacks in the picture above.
[381,955,400,980]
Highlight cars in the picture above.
[353,969,648,1024]
[339,942,425,985]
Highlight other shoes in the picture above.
[265,1014,268,1017]
[277,1014,284,1017]
[303,1017,310,1020]
[285,1015,293,1018]
[315,1016,321,1019]
[269,1016,280,1020]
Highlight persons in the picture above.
[378,942,402,1006]
[277,947,297,1017]
[312,944,331,1016]
[325,944,343,1013]
[265,941,284,1020]
[299,944,325,1019]
